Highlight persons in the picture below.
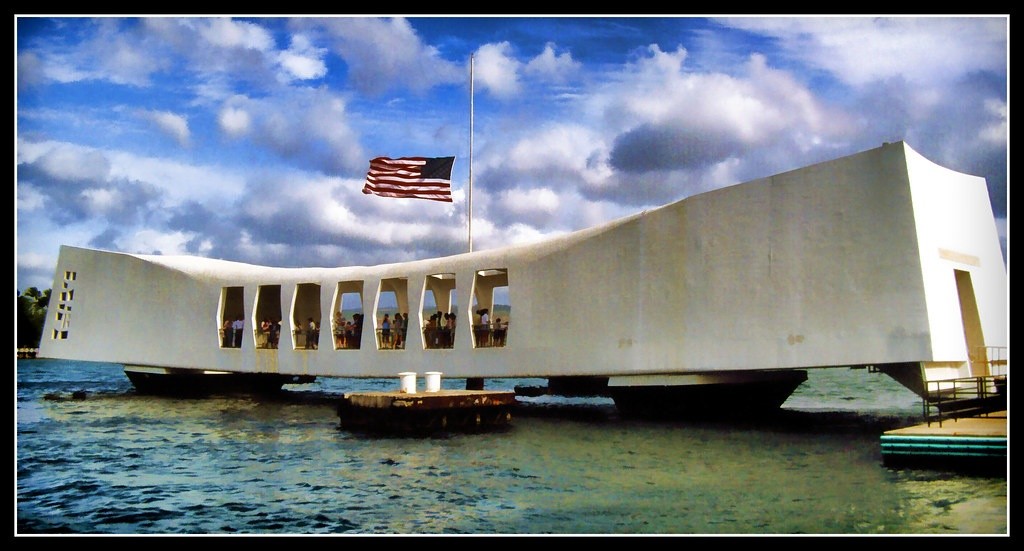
[381,313,408,349]
[223,317,244,348]
[473,309,508,347]
[335,313,363,349]
[424,311,456,349]
[259,316,282,349]
[295,317,320,349]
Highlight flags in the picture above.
[362,155,455,202]
